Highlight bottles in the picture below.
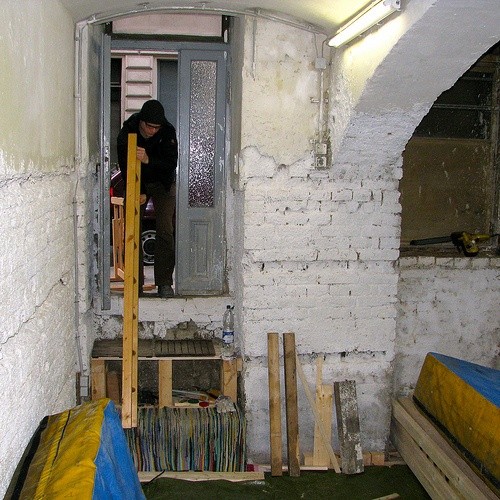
[222,305,235,360]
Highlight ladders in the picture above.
[120,135,141,430]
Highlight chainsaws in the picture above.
[410,231,490,256]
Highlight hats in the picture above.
[139,99,165,125]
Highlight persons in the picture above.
[116,100,178,298]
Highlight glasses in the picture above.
[145,122,161,129]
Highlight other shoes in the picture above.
[138,287,143,298]
[158,285,174,298]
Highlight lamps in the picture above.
[326,0,402,49]
